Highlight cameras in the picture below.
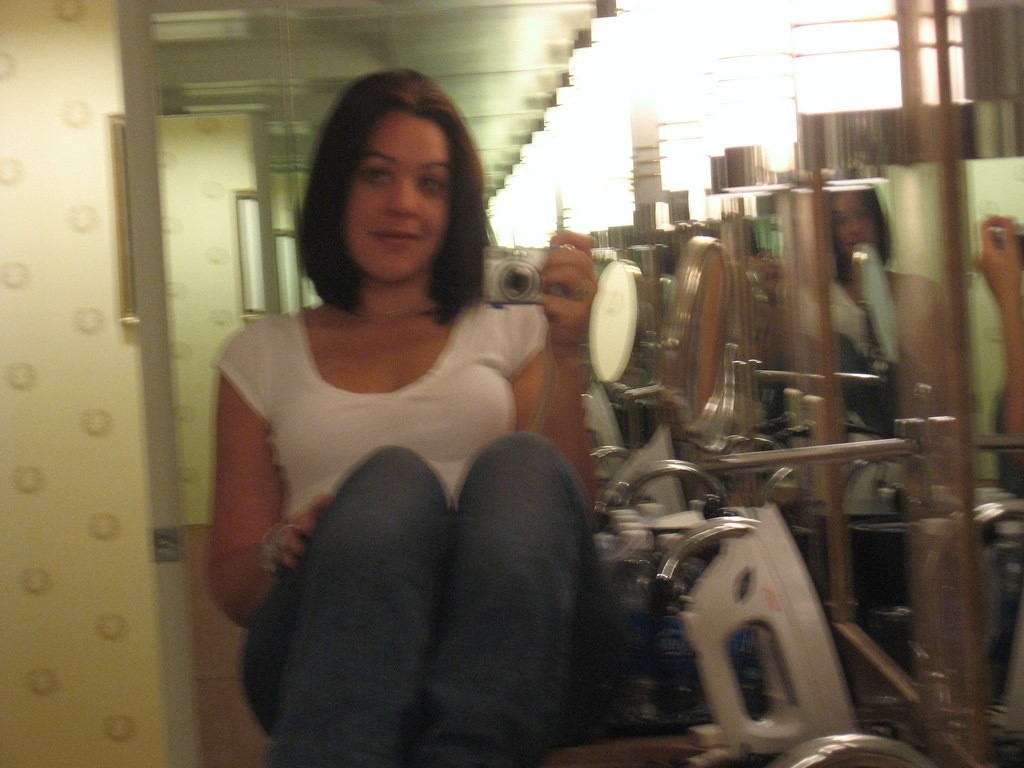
[482,245,566,304]
[991,229,1024,271]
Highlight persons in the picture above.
[979,215,1023,496]
[830,187,945,442]
[205,69,602,768]
